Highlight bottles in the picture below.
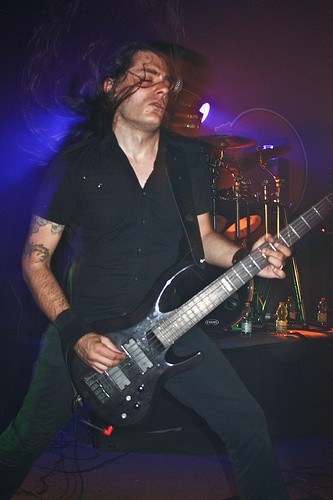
[286,296,295,325]
[241,302,252,337]
[317,297,327,325]
[276,300,288,333]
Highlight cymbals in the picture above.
[247,146,291,161]
[196,134,255,150]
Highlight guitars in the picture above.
[65,189,333,430]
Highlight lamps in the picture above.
[179,87,211,124]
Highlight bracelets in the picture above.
[232,248,251,266]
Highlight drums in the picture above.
[213,188,263,242]
[215,158,242,202]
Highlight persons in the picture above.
[0,41,291,500]
